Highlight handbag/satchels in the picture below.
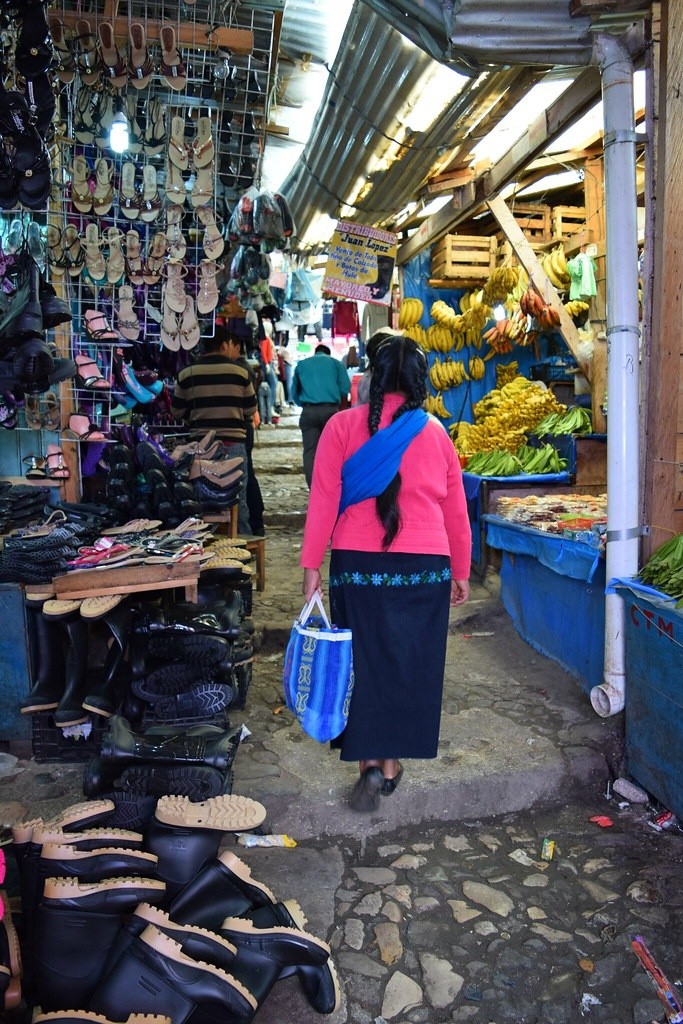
[283,589,355,744]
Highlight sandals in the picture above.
[0,0,224,390]
[22,391,105,480]
[0,510,85,584]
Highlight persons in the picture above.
[291,344,352,493]
[347,346,359,369]
[356,332,393,409]
[258,382,271,426]
[169,324,265,537]
[259,321,298,418]
[300,331,472,812]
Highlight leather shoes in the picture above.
[0,341,78,395]
[7,264,72,338]
[44,425,245,529]
[351,761,404,812]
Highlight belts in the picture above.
[301,403,338,407]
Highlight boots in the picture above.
[0,539,340,1023]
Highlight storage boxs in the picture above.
[480,201,586,267]
[530,362,579,381]
[430,235,497,281]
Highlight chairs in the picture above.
[203,503,267,592]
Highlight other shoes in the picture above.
[218,66,293,311]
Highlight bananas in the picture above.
[399,245,589,419]
[447,359,568,459]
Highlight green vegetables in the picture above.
[528,405,596,437]
[463,441,567,476]
[635,530,683,610]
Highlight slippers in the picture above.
[67,516,217,566]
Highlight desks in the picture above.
[479,514,606,695]
